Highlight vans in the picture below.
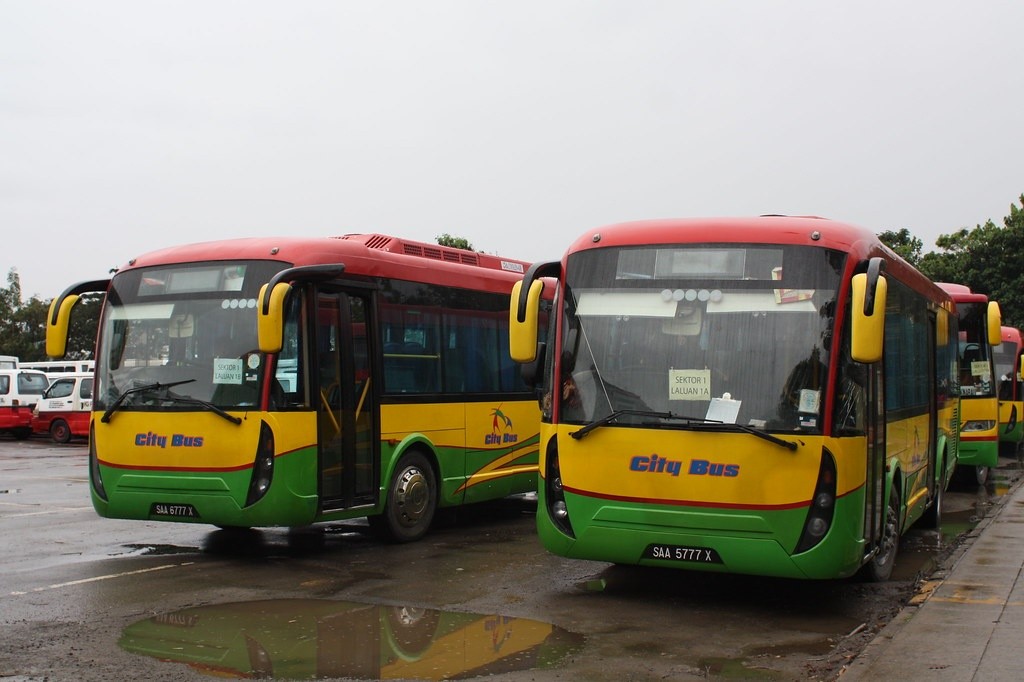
[0,352,105,448]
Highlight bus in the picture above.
[46,234,548,543]
[505,202,1024,594]
